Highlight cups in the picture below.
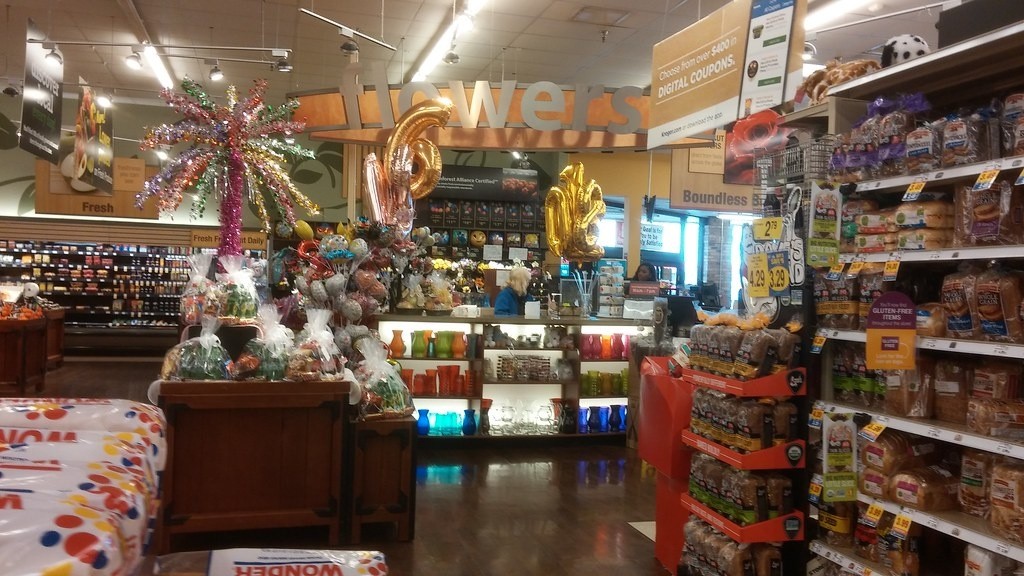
[437,365,459,396]
[454,304,481,318]
[599,266,624,315]
[430,412,462,435]
[401,369,438,396]
[460,370,477,396]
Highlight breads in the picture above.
[806,187,1024,251]
[808,96,1024,182]
[683,521,786,576]
[691,327,802,378]
[850,428,1024,544]
[823,499,1024,576]
[833,349,1024,442]
[811,264,1024,342]
[696,458,792,526]
[691,389,798,454]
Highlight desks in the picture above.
[0,309,66,397]
[150,379,348,554]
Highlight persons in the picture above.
[494,267,532,317]
[632,264,656,282]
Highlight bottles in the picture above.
[579,405,628,433]
[581,333,630,360]
[580,368,628,397]
[417,409,430,435]
[462,410,476,435]
[490,403,566,435]
[467,333,483,358]
[389,330,404,358]
[412,329,466,358]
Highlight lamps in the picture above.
[45,43,63,69]
[442,0,460,65]
[340,27,360,57]
[127,48,143,72]
[209,64,223,81]
[270,57,293,73]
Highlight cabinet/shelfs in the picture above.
[376,314,656,442]
[679,21,1024,576]
[0,238,190,349]
[347,411,418,546]
